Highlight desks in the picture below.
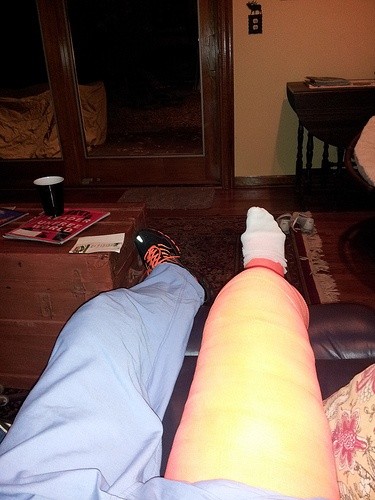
[285,77,375,212]
[0,201,150,392]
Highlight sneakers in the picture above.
[135,229,214,305]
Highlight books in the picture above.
[0,208,29,227]
[69,233,125,254]
[2,208,111,244]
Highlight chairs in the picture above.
[151,298,375,469]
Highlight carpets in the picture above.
[145,214,340,305]
[117,187,218,210]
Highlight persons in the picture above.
[0,206,341,500]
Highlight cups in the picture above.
[33,176,66,217]
